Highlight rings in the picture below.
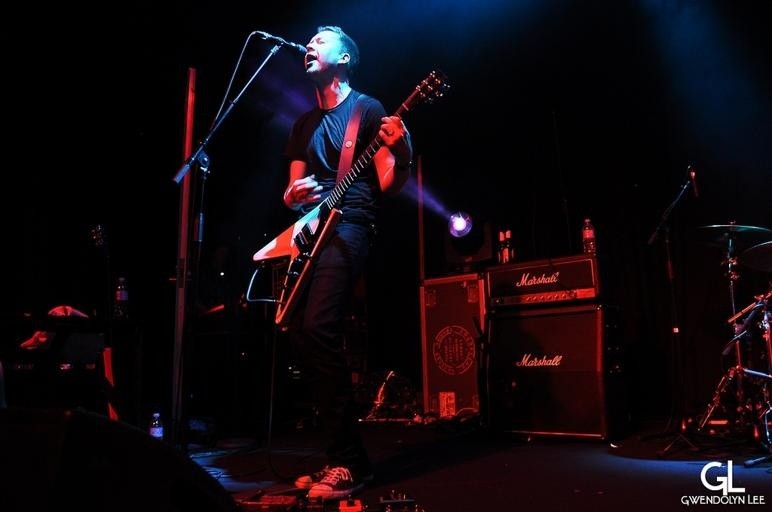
[388,131,395,136]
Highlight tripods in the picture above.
[695,237,772,453]
[636,184,710,454]
[439,313,543,451]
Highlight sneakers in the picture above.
[294,464,330,489]
[307,468,363,497]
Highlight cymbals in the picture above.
[721,241,772,282]
[699,225,769,231]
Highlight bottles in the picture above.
[113,277,129,318]
[496,225,516,266]
[581,219,597,257]
[149,413,164,441]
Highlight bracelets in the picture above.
[396,159,417,170]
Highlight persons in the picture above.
[277,24,415,501]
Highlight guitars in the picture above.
[253,70,450,324]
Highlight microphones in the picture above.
[690,166,700,198]
[257,30,307,56]
